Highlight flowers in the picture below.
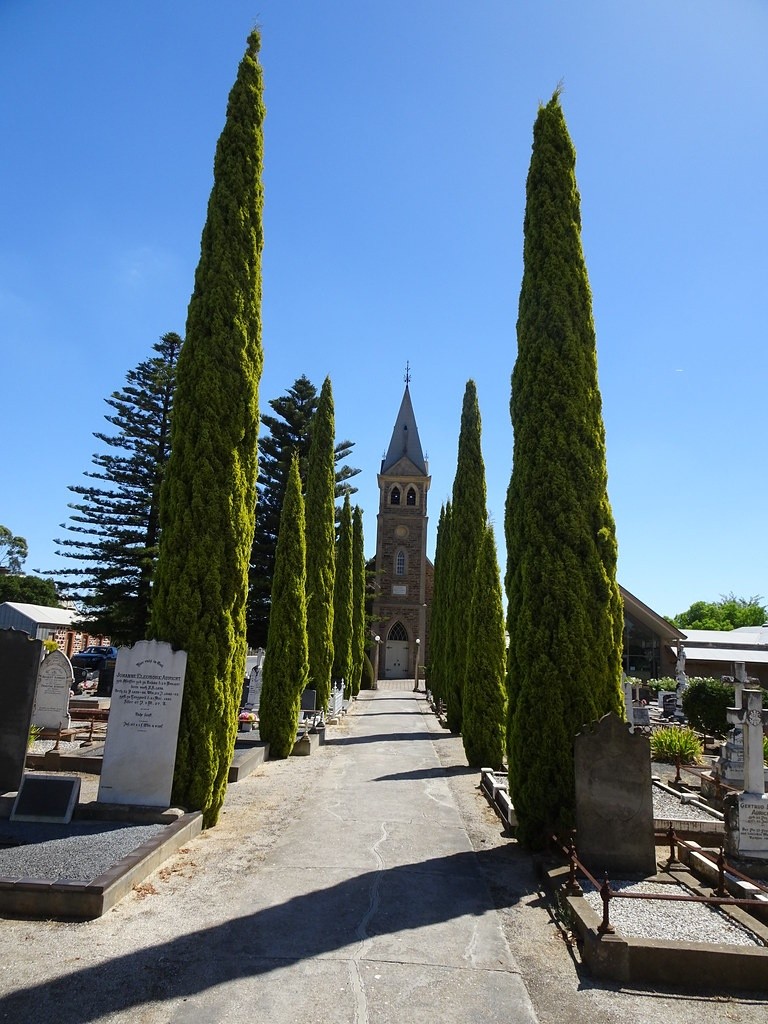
[239,712,256,722]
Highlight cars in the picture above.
[70,645,118,670]
